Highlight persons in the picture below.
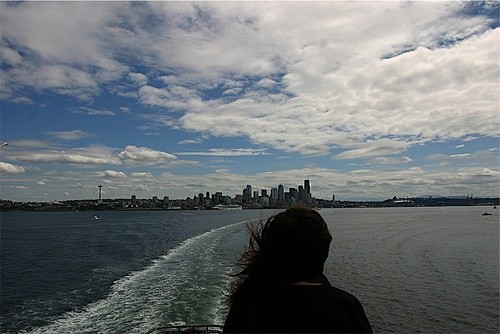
[92,214,101,220]
[224,205,374,334]
